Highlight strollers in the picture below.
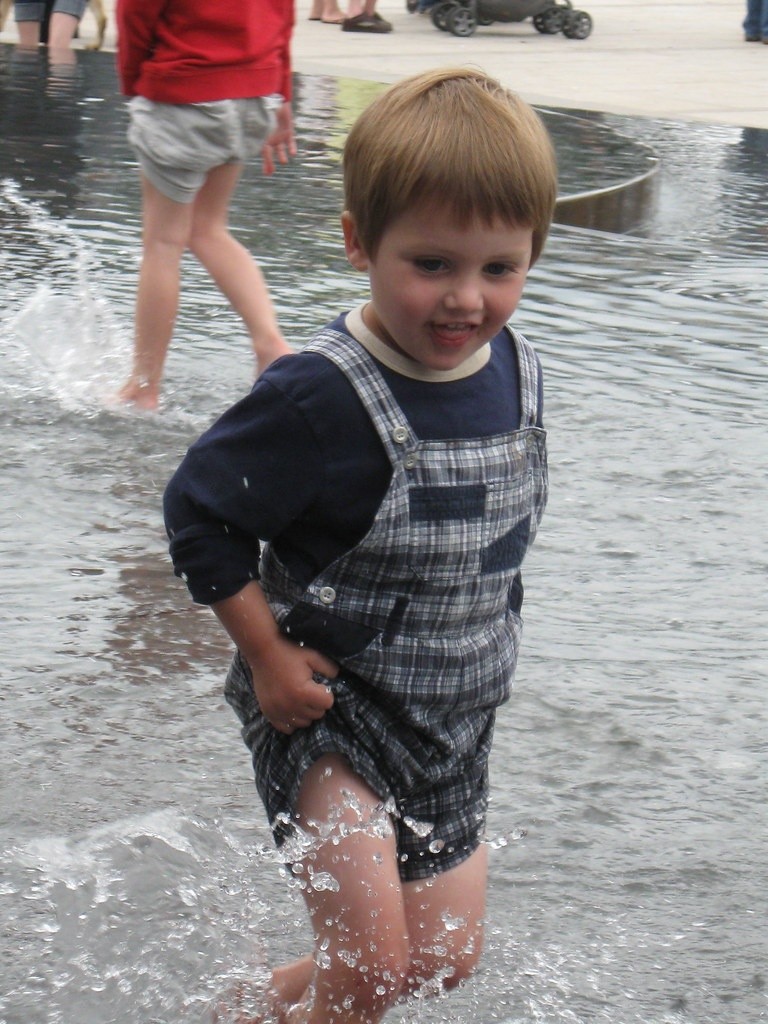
[430,0,593,40]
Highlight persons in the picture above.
[742,0,768,44]
[115,0,298,413]
[1,0,392,63]
[164,70,557,1024]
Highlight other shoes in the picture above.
[342,10,393,33]
